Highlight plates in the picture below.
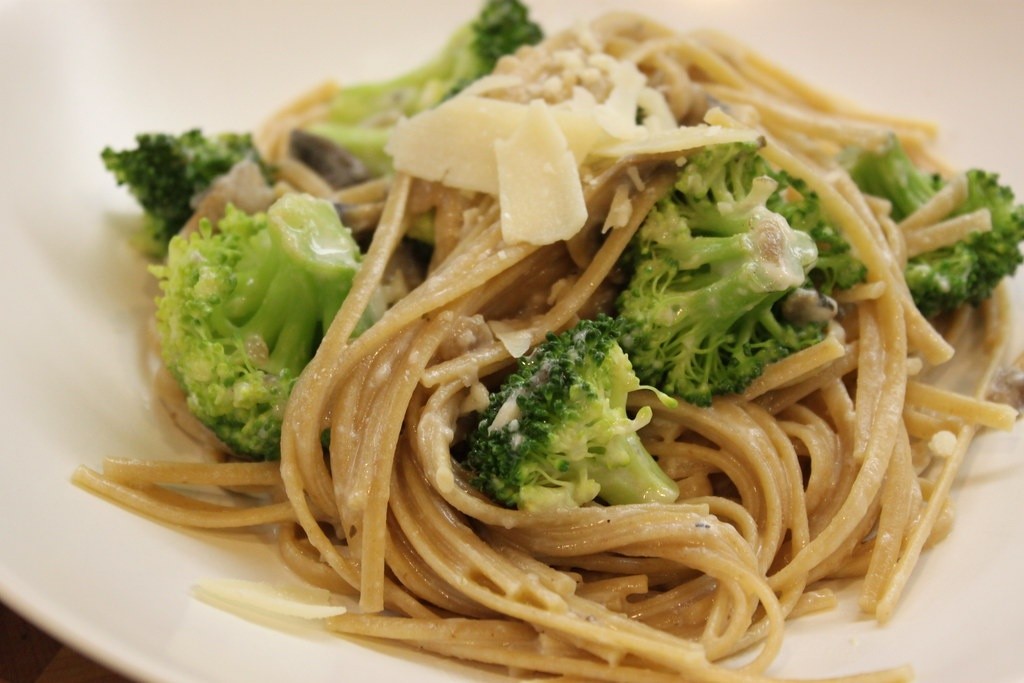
[0,4,1024,682]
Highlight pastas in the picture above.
[68,10,1021,683]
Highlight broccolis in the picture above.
[104,0,1024,505]
